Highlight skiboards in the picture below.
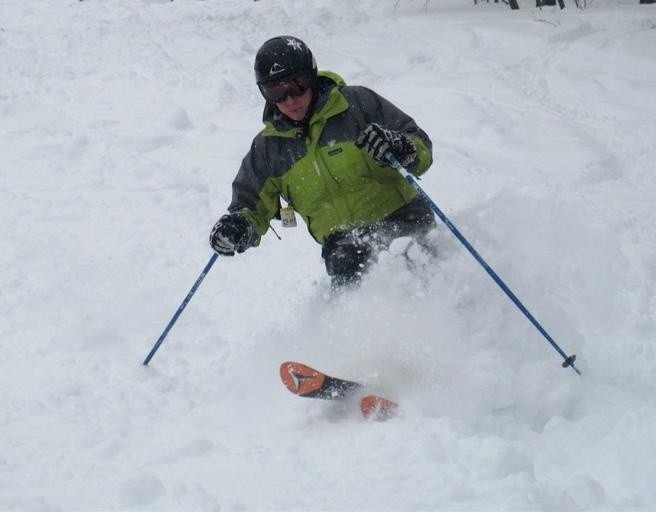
[280,361,405,423]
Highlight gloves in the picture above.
[355,121,417,169]
[209,212,254,257]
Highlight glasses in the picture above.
[257,70,313,103]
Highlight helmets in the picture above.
[253,34,318,104]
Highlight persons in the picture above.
[208,37,434,297]
